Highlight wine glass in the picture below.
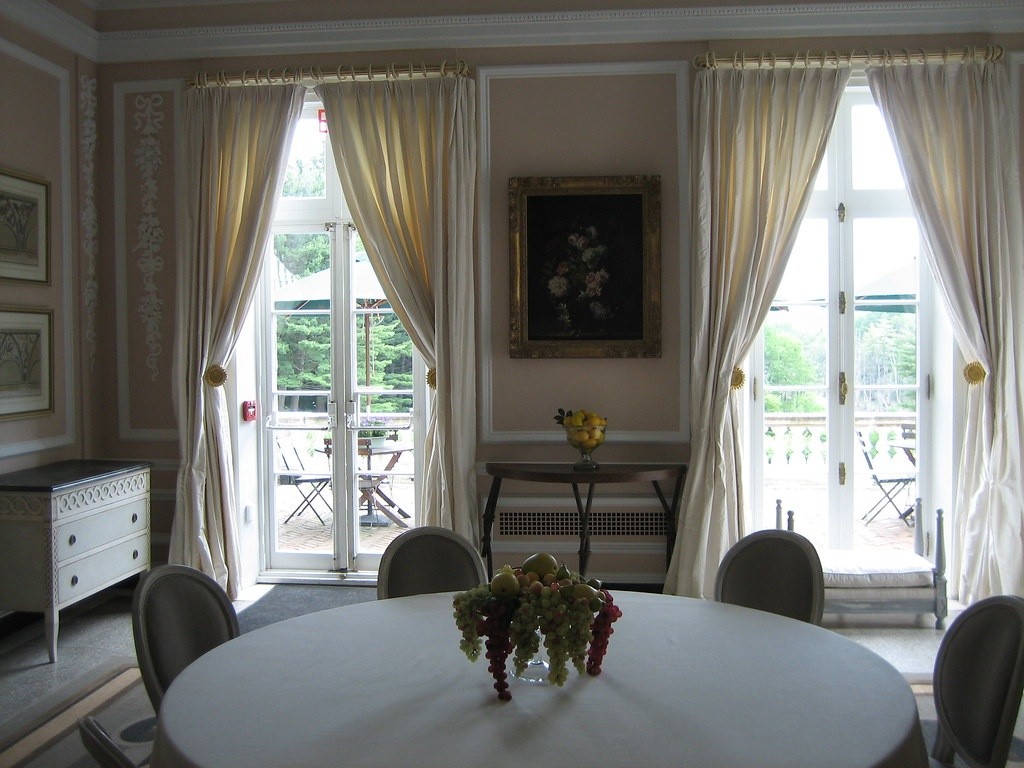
[565,425,607,471]
[509,628,551,682]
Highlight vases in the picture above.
[358,436,387,449]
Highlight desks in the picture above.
[480,461,687,593]
[318,436,416,528]
[150,588,931,768]
[889,438,918,520]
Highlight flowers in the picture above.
[356,414,393,433]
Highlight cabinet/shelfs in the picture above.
[0,459,156,661]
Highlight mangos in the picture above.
[559,583,597,602]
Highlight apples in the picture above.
[520,552,558,578]
[491,572,520,598]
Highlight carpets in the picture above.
[0,654,159,768]
[236,582,379,637]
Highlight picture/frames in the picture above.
[1,165,53,288]
[508,173,663,360]
[0,303,56,423]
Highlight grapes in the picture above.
[453,564,622,702]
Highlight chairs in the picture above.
[854,422,916,529]
[272,429,400,536]
[775,497,948,631]
[76,524,1022,768]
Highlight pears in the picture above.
[563,409,607,447]
